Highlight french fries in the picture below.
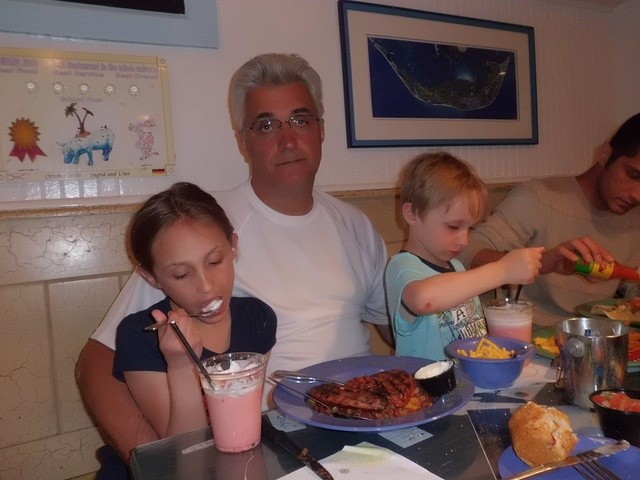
[454,337,520,361]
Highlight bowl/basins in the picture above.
[443,335,537,388]
[414,360,456,396]
[589,389,640,446]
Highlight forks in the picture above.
[268,376,373,425]
[572,455,624,480]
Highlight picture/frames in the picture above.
[0,0,221,49]
[0,47,176,180]
[336,0,540,148]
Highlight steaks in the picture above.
[345,368,416,406]
[304,383,387,418]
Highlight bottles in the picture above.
[562,252,640,284]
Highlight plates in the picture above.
[497,433,639,476]
[577,296,638,321]
[271,353,477,432]
[530,325,639,367]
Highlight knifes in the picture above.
[500,438,632,480]
[259,414,338,480]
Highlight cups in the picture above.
[483,298,535,345]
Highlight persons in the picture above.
[73,52,391,469]
[454,112,640,329]
[384,150,545,362]
[91,181,276,480]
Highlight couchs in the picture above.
[0,181,520,480]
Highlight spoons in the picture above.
[144,300,223,330]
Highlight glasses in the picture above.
[242,115,320,138]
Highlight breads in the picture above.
[507,402,579,469]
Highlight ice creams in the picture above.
[482,297,535,368]
